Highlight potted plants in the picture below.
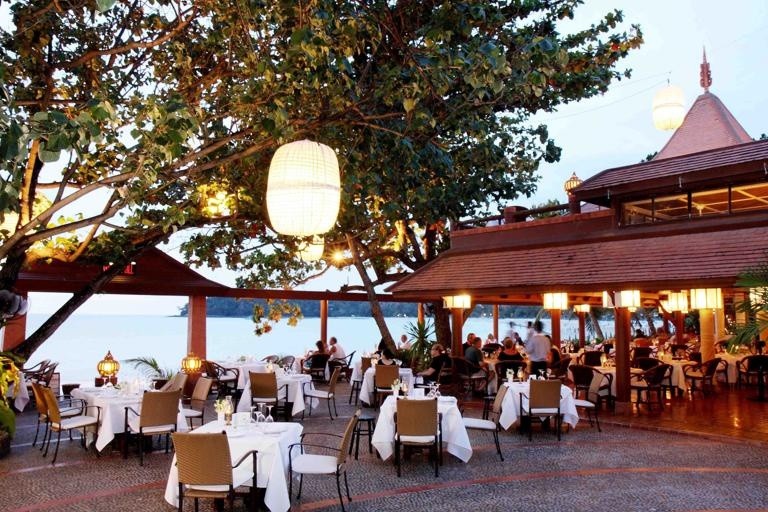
[126,354,173,389]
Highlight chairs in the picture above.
[393,397,443,478]
[125,387,182,466]
[158,374,213,452]
[32,382,103,463]
[170,431,258,512]
[0,359,60,415]
[288,408,362,512]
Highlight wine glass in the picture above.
[101,377,108,387]
[538,367,551,380]
[150,381,158,391]
[284,363,296,377]
[427,381,442,400]
[249,402,275,428]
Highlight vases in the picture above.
[63,384,80,399]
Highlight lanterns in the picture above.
[300,235,325,263]
[266,138,341,237]
[653,87,684,132]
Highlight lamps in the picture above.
[443,294,471,310]
[614,290,640,309]
[97,350,120,385]
[544,294,567,311]
[690,288,722,310]
[658,300,672,313]
[668,293,688,312]
[574,304,590,312]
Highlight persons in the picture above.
[374,321,563,385]
[636,329,645,338]
[329,337,346,366]
[309,340,330,355]
